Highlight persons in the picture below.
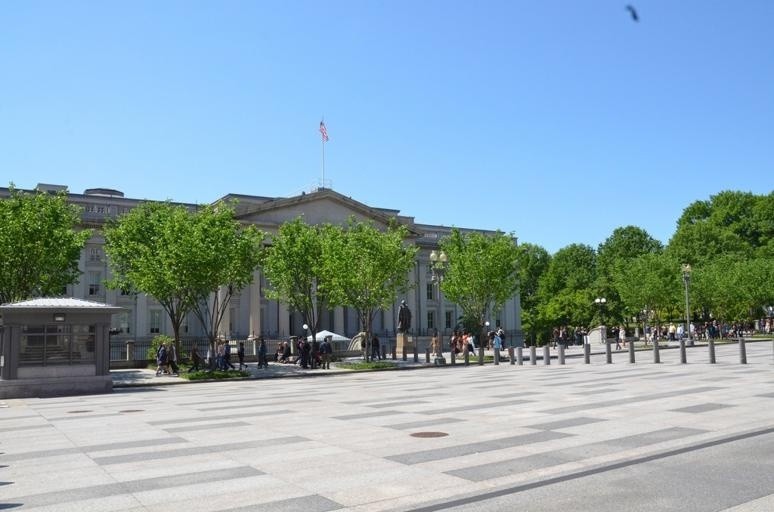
[646,318,766,342]
[361,333,381,360]
[258,338,268,369]
[574,326,588,345]
[613,325,626,351]
[430,326,506,362]
[552,325,568,349]
[238,342,248,370]
[274,335,332,369]
[155,339,179,377]
[187,339,235,373]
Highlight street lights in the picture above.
[682,264,694,346]
[640,309,653,346]
[595,298,606,325]
[430,250,447,365]
[485,321,490,350]
[303,322,309,337]
